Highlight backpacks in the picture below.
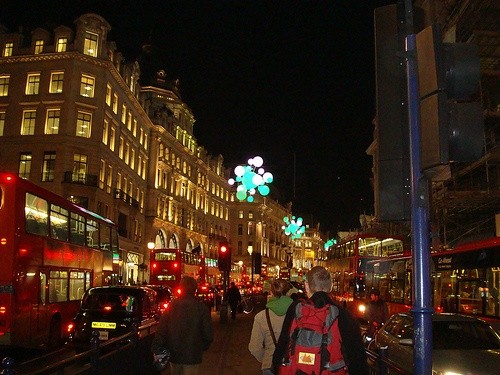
[278,298,350,375]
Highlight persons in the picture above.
[250,279,293,375]
[228,282,241,318]
[151,276,215,375]
[271,266,368,375]
[368,289,388,326]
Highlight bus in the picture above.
[362,236,500,336]
[325,228,440,313]
[148,248,206,291]
[0,170,125,351]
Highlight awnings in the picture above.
[367,238,500,263]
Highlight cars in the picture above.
[65,284,160,367]
[363,309,500,375]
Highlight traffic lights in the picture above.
[217,244,232,272]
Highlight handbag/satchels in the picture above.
[237,301,246,314]
